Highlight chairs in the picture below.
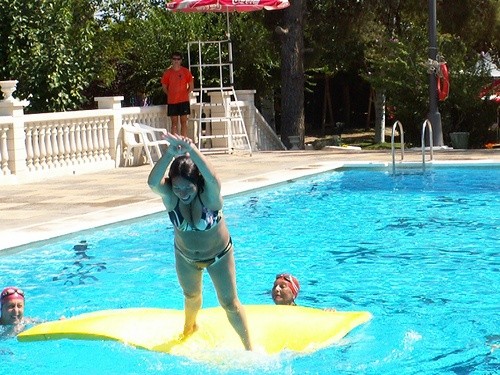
[121,123,173,168]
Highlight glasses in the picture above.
[0,289,24,301]
[276,274,292,282]
[172,58,181,60]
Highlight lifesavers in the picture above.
[436,60,450,102]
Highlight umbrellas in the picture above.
[476,79,500,143]
[166,0,291,91]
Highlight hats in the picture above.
[275,274,299,297]
[0,287,25,303]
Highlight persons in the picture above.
[271,272,301,307]
[135,87,153,106]
[146,133,255,354]
[160,51,194,149]
[0,287,67,340]
